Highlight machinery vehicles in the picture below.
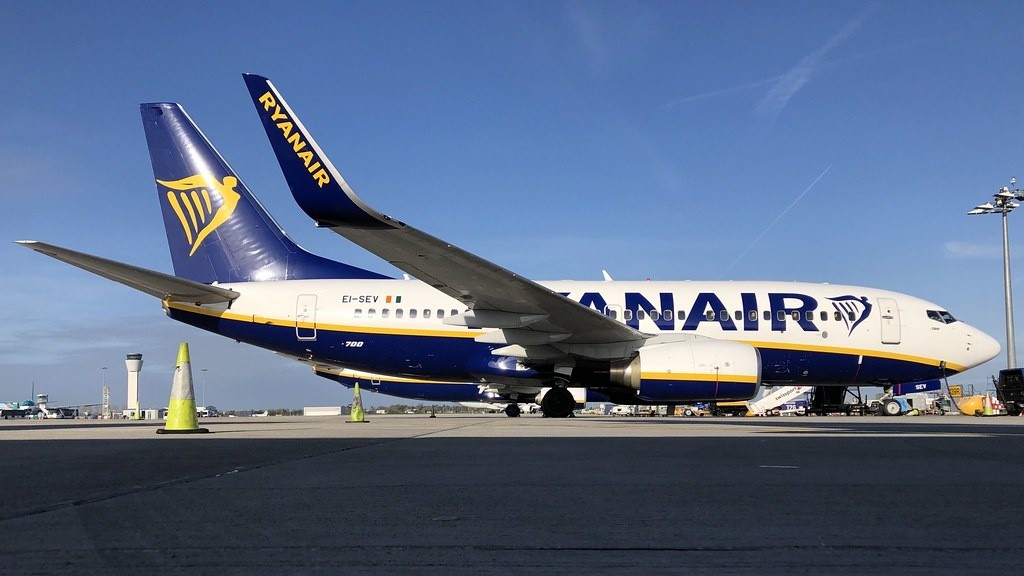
[747,386,813,417]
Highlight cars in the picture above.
[197,409,217,417]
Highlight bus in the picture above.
[0,407,79,419]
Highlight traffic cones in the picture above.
[982,391,996,417]
[132,400,144,420]
[344,382,370,423]
[156,342,209,434]
[429,405,437,418]
[906,408,919,416]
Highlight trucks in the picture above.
[612,404,712,417]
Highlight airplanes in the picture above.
[14,73,1001,417]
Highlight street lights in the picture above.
[968,179,1024,369]
[200,368,208,408]
[103,366,108,387]
